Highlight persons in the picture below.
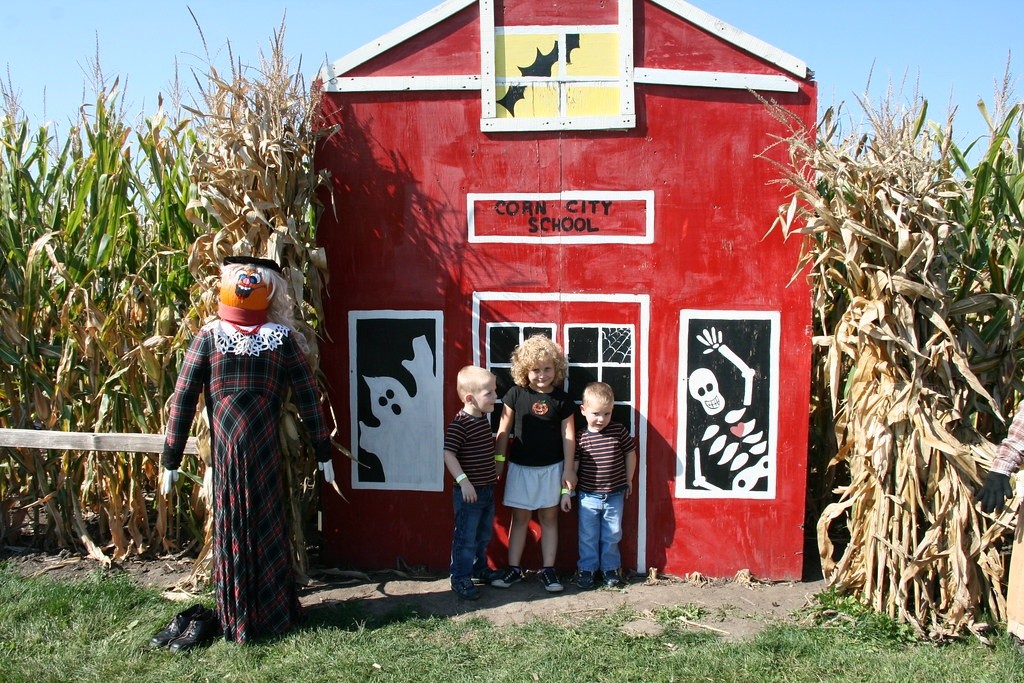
[491,330,578,594]
[561,380,639,588]
[159,255,338,643]
[440,365,502,599]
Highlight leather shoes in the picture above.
[148,603,205,649]
[169,608,221,655]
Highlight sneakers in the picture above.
[471,567,506,586]
[540,567,564,592]
[576,570,595,589]
[601,569,621,587]
[451,576,481,600]
[491,567,522,588]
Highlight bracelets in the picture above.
[493,455,508,462]
[456,473,468,485]
[560,488,572,496]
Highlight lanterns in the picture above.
[217,265,278,311]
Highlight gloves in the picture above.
[159,467,179,495]
[318,458,335,484]
